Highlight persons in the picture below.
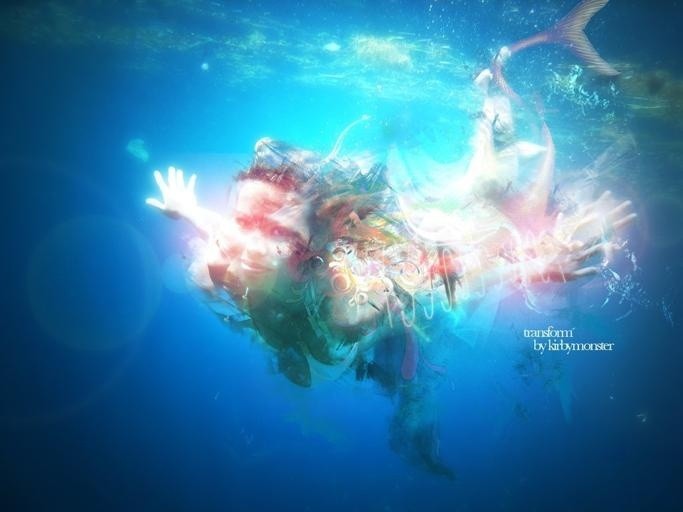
[143,135,638,485]
